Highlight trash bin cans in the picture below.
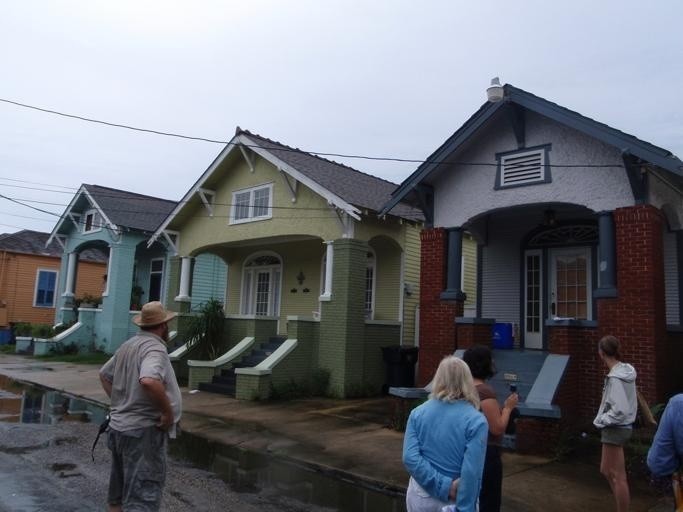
[0,326,11,344]
[493,323,513,349]
[381,345,419,395]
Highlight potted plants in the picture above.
[73,293,101,308]
[13,322,56,339]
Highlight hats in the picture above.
[132,301,177,325]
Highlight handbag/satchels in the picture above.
[631,391,657,431]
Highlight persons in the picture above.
[462,344,519,511]
[647,392,683,512]
[96,298,185,512]
[399,353,492,512]
[593,335,641,512]
